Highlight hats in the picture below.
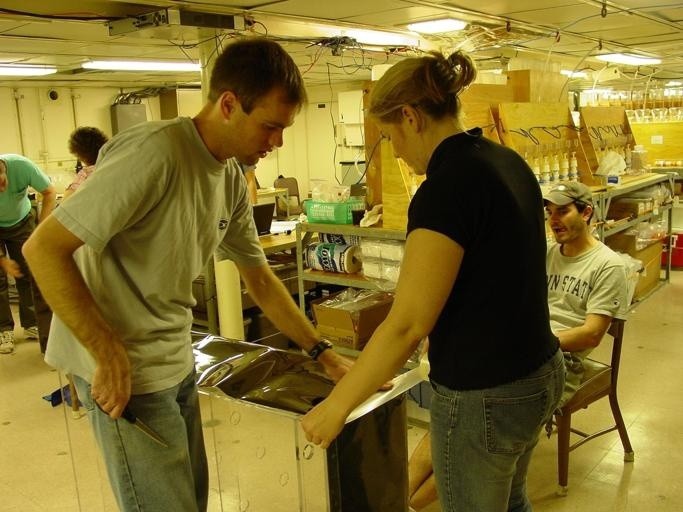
[543,180,594,208]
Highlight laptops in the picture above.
[253,202,276,236]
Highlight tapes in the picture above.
[345,352,431,424]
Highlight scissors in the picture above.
[95,400,168,447]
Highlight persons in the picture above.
[300,48,566,512]
[61,125,111,203]
[407,181,628,511]
[20,37,394,512]
[0,153,57,356]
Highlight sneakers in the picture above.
[23,326,39,340]
[0,330,15,354]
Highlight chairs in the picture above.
[543,316,635,496]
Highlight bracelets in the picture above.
[0,254,5,260]
[307,337,334,362]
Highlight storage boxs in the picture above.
[309,287,396,349]
[606,220,663,309]
